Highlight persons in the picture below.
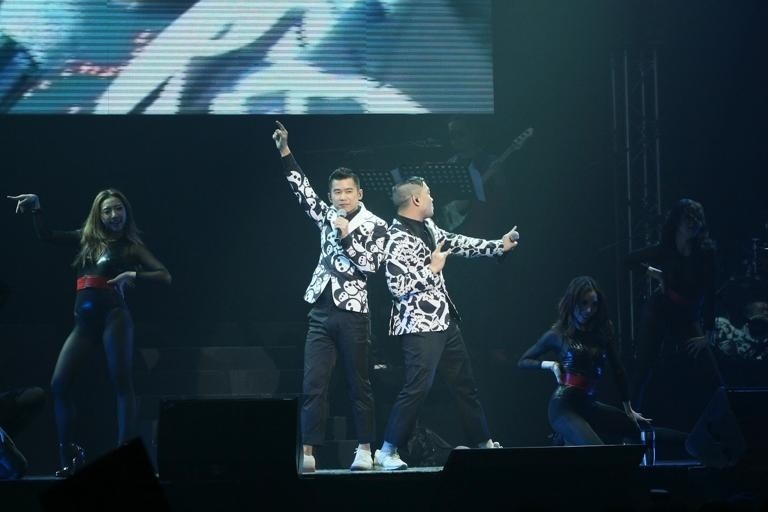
[374,176,520,469]
[623,194,754,466]
[5,188,174,478]
[518,276,648,446]
[272,120,391,471]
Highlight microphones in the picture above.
[335,209,346,240]
[497,231,519,262]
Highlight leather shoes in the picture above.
[54,442,88,476]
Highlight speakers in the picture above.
[443,444,648,512]
[684,386,768,468]
[159,398,301,476]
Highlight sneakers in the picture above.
[351,450,372,470]
[302,453,316,472]
[373,447,408,470]
